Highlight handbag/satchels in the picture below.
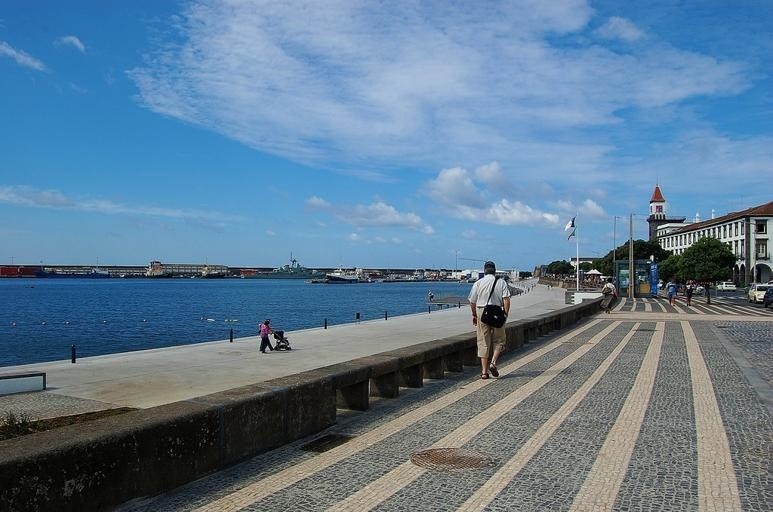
[481,305,507,329]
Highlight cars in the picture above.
[744,280,773,308]
[657,279,706,295]
[716,281,736,292]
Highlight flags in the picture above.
[565,213,575,230]
[568,225,577,241]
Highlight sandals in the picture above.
[489,363,499,377]
[481,374,489,379]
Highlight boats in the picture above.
[306,264,509,283]
[201,267,219,278]
[144,260,173,278]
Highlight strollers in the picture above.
[274,331,291,351]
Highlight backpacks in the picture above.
[602,284,612,294]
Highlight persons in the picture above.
[258,320,270,351]
[600,278,618,314]
[666,279,676,307]
[684,279,693,306]
[467,261,511,380]
[261,321,275,353]
[428,289,435,303]
